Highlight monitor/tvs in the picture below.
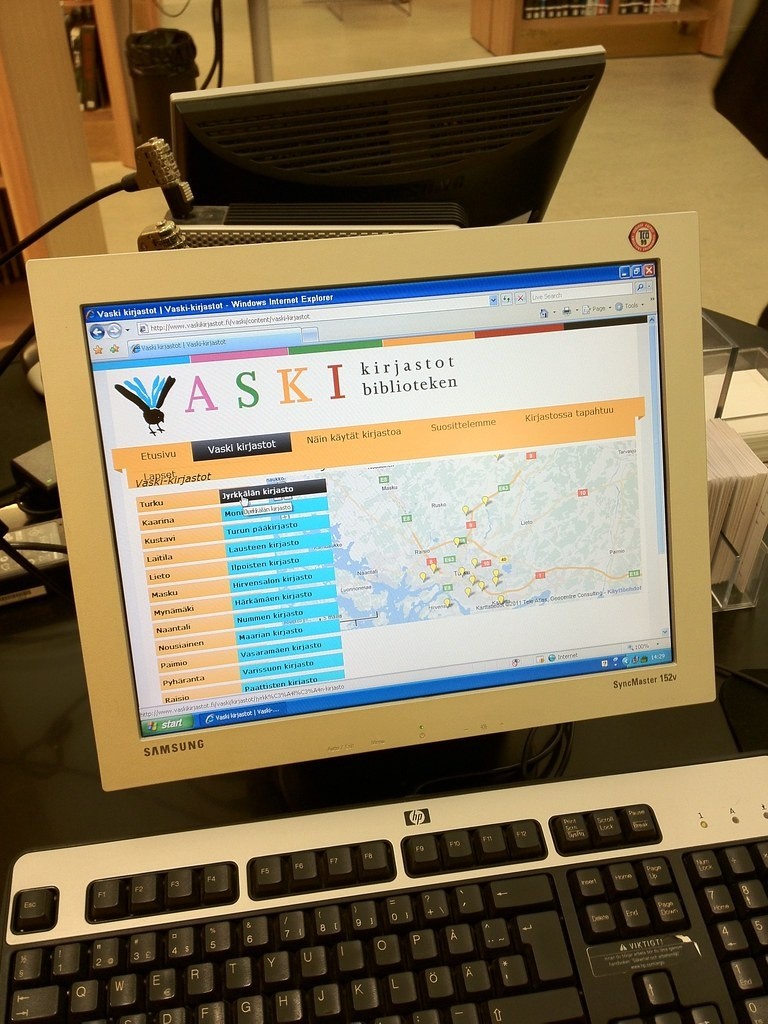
[25,211,718,812]
[169,45,608,225]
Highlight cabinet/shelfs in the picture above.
[0,1,157,362]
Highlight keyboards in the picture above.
[0,754,768,1024]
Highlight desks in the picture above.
[0,307,768,969]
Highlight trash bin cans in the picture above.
[127,28,197,143]
